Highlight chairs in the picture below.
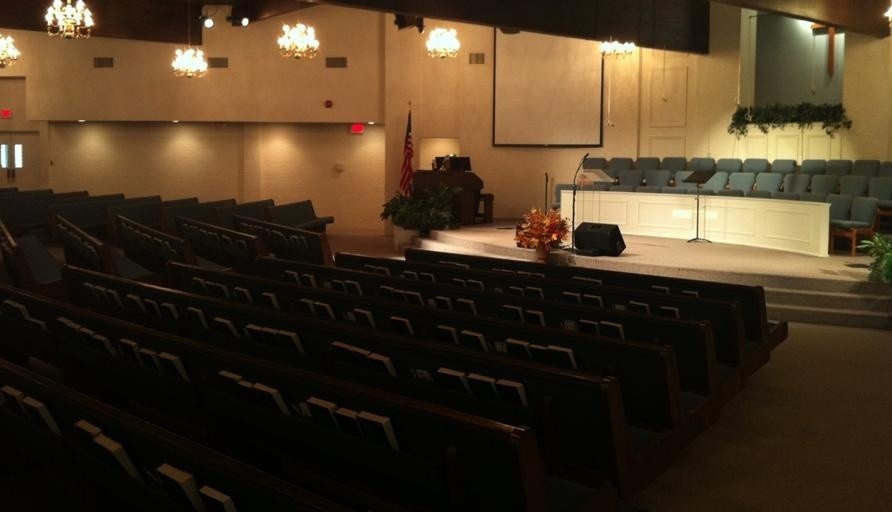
[1,185,787,510]
[549,157,891,255]
[433,156,494,223]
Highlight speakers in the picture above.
[574,221,626,256]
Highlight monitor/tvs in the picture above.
[435,156,472,171]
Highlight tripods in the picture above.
[687,183,712,243]
[562,160,596,254]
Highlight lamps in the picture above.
[169,5,320,80]
[598,32,636,58]
[426,16,460,60]
[0,0,95,67]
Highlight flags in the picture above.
[399,99,415,200]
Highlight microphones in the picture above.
[584,153,589,160]
[544,172,549,183]
